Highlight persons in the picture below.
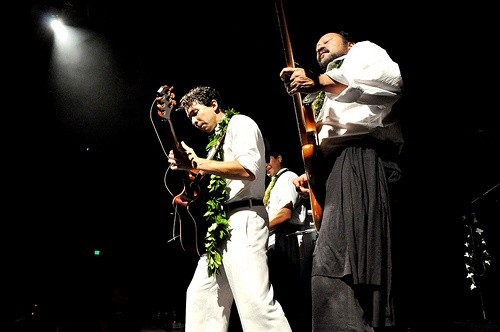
[279,29,405,332]
[168,87,306,332]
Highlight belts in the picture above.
[224,199,264,212]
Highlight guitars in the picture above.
[273,0,325,231]
[157,84,212,257]
